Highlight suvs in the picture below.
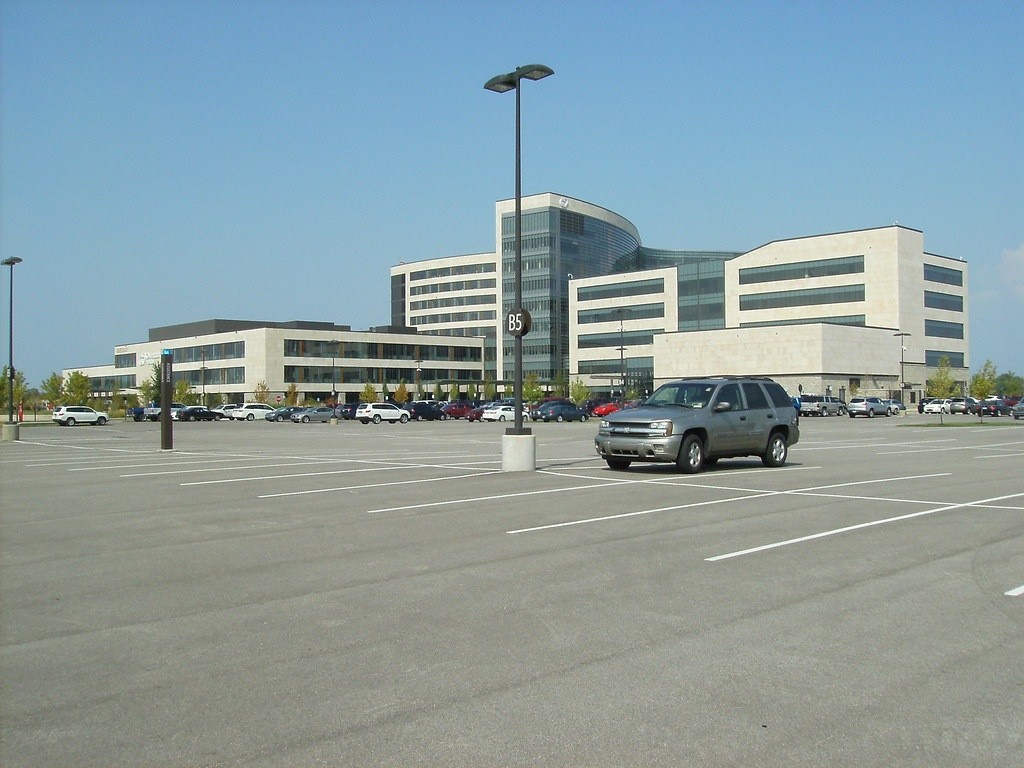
[52,406,110,426]
[355,402,412,425]
[594,374,800,475]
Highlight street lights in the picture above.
[0,256,22,425]
[893,331,911,408]
[610,307,633,411]
[480,64,558,477]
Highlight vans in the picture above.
[799,395,844,416]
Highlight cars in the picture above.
[399,402,447,421]
[126,402,209,422]
[880,399,906,414]
[290,406,334,423]
[324,401,361,420]
[1012,397,1024,419]
[917,396,937,414]
[265,407,307,423]
[845,396,891,418]
[967,393,1022,407]
[788,397,801,411]
[211,403,237,420]
[175,406,225,422]
[949,397,977,416]
[400,397,649,423]
[923,399,953,415]
[974,400,1012,418]
[231,402,278,421]
